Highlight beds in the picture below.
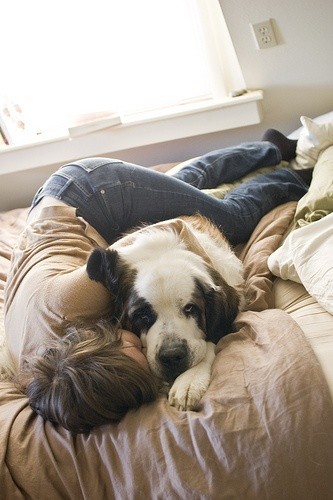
[0,106,333,500]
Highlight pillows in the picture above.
[294,144,333,229]
[291,116,333,171]
[267,211,333,315]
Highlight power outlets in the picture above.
[250,17,278,50]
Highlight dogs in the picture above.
[85,211,244,411]
